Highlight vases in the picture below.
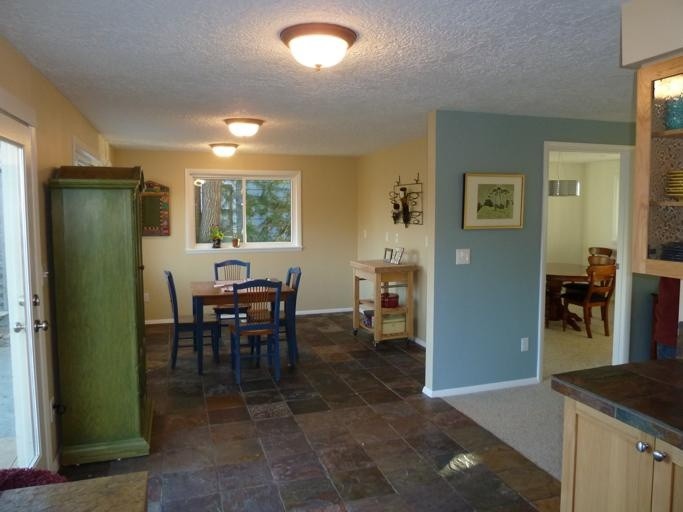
[213,239,220,248]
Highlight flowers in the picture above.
[208,224,224,240]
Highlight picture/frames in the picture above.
[463,173,525,229]
[384,248,393,263]
[391,247,404,264]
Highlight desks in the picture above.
[546,261,589,321]
[350,260,420,347]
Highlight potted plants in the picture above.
[232,234,239,247]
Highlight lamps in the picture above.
[209,144,238,158]
[280,23,357,69]
[223,118,264,138]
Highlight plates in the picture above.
[659,240,683,262]
[664,170,683,202]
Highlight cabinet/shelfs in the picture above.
[49,165,155,467]
[551,357,681,511]
[632,52,683,280]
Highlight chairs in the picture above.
[163,260,301,384]
[561,246,616,339]
